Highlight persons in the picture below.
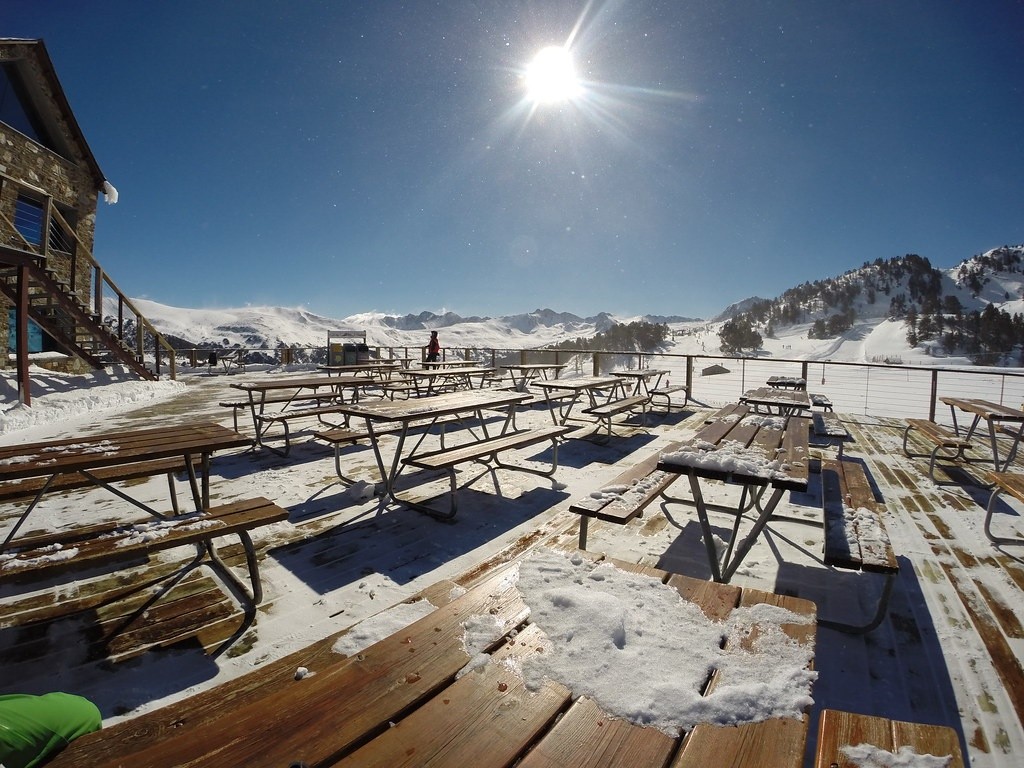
[425,331,440,371]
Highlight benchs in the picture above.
[569,441,686,551]
[0,497,289,605]
[581,396,651,446]
[903,418,972,486]
[705,404,751,425]
[992,424,1024,465]
[814,710,964,768]
[205,364,213,376]
[220,367,583,522]
[812,411,848,474]
[984,472,1024,545]
[237,361,245,374]
[647,385,689,416]
[588,382,634,407]
[41,579,470,768]
[0,453,213,517]
[820,453,900,635]
[809,394,833,448]
[738,390,756,413]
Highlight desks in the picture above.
[96,542,816,768]
[228,376,375,447]
[939,397,1024,490]
[219,356,236,375]
[399,367,497,398]
[500,364,567,393]
[316,364,402,404]
[0,422,252,561]
[417,360,483,383]
[530,376,626,440]
[657,411,809,584]
[359,359,417,384]
[766,376,806,417]
[746,387,810,417]
[609,370,671,414]
[338,389,534,503]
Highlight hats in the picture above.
[431,331,437,334]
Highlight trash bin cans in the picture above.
[356,343,369,365]
[329,343,344,366]
[209,352,217,364]
[343,343,357,365]
